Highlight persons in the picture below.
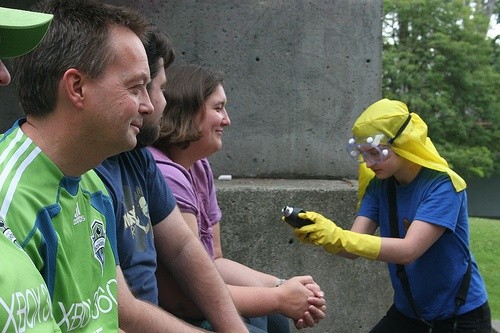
[146,61,326,333]
[93,24,251,333]
[0,8,62,333]
[0,0,124,333]
[282,97,491,333]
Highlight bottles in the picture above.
[281,206,315,237]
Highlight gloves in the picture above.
[282,206,381,264]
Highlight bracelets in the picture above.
[276,279,286,287]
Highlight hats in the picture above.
[0,6,54,59]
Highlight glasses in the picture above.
[344,115,414,164]
